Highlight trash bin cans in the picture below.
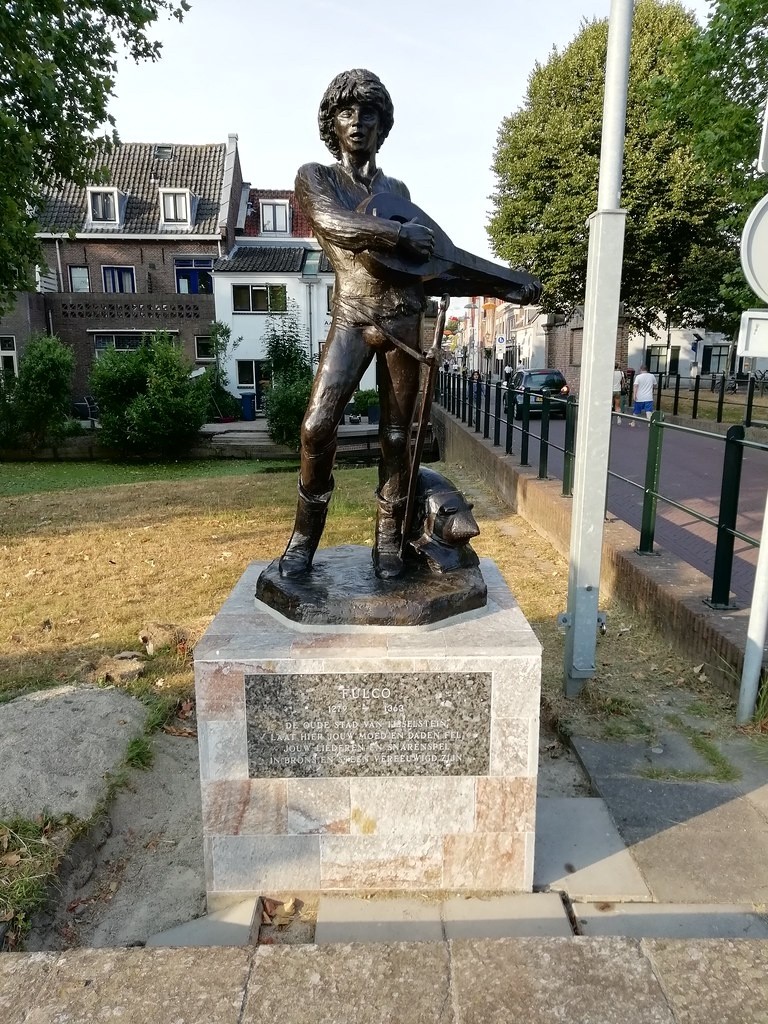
[239,392,256,421]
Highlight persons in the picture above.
[628,365,657,428]
[504,364,513,384]
[466,371,485,424]
[444,362,449,373]
[278,69,543,583]
[453,363,458,371]
[613,362,625,426]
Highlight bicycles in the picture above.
[712,376,738,395]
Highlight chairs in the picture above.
[84,395,103,427]
[545,375,555,387]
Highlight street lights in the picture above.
[693,333,703,362]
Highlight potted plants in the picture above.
[219,393,242,423]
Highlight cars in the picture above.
[503,368,576,420]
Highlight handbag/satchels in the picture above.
[621,372,628,396]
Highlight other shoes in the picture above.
[629,421,635,427]
[617,417,621,425]
[648,425,651,429]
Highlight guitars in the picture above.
[351,191,541,290]
